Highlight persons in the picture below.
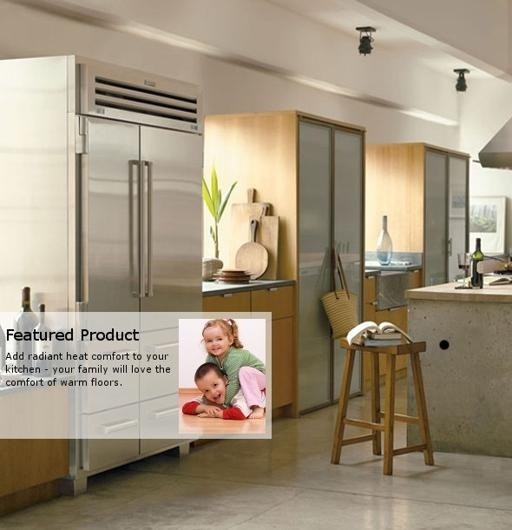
[181,318,269,420]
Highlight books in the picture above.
[346,318,415,348]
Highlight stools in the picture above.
[329,339,434,476]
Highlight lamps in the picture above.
[453,68,470,92]
[355,26,377,56]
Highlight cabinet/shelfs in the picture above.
[0,384,72,517]
[203,109,368,416]
[362,269,425,393]
[193,284,297,448]
[364,142,471,286]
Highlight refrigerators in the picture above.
[0,56,204,477]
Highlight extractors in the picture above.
[478,116,512,169]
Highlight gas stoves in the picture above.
[493,268,512,274]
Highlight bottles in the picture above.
[471,238,483,286]
[375,216,393,266]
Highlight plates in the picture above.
[212,270,252,282]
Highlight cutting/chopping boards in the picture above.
[224,188,279,280]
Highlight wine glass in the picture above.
[457,252,470,288]
